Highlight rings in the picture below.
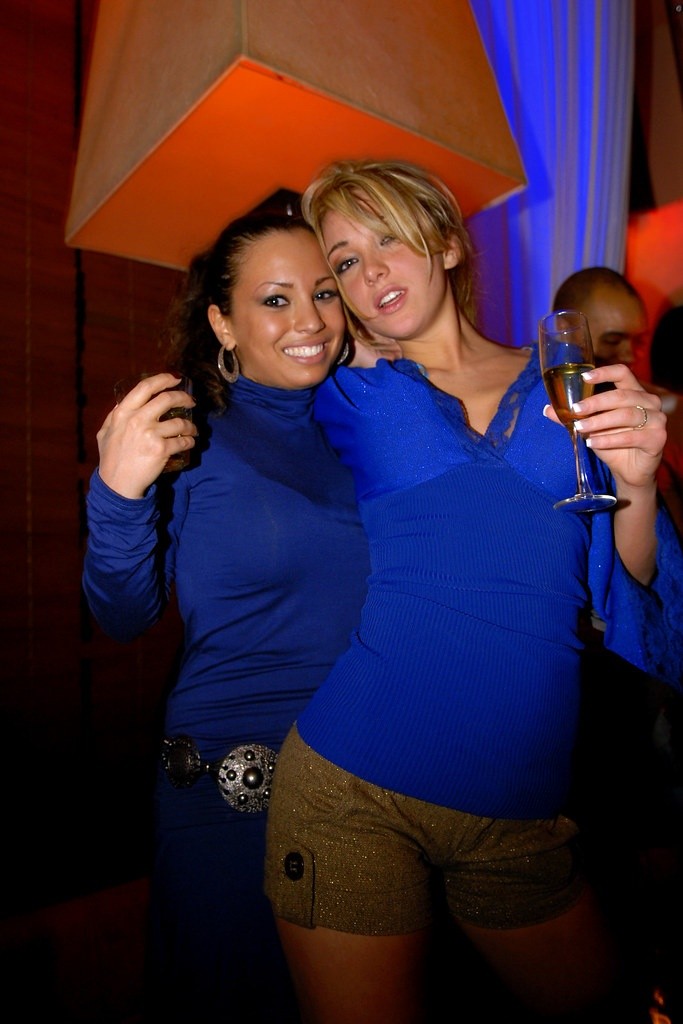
[634,405,648,428]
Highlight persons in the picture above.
[261,158,683,1024]
[86,194,384,1024]
[547,264,683,830]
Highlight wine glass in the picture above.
[537,310,617,515]
